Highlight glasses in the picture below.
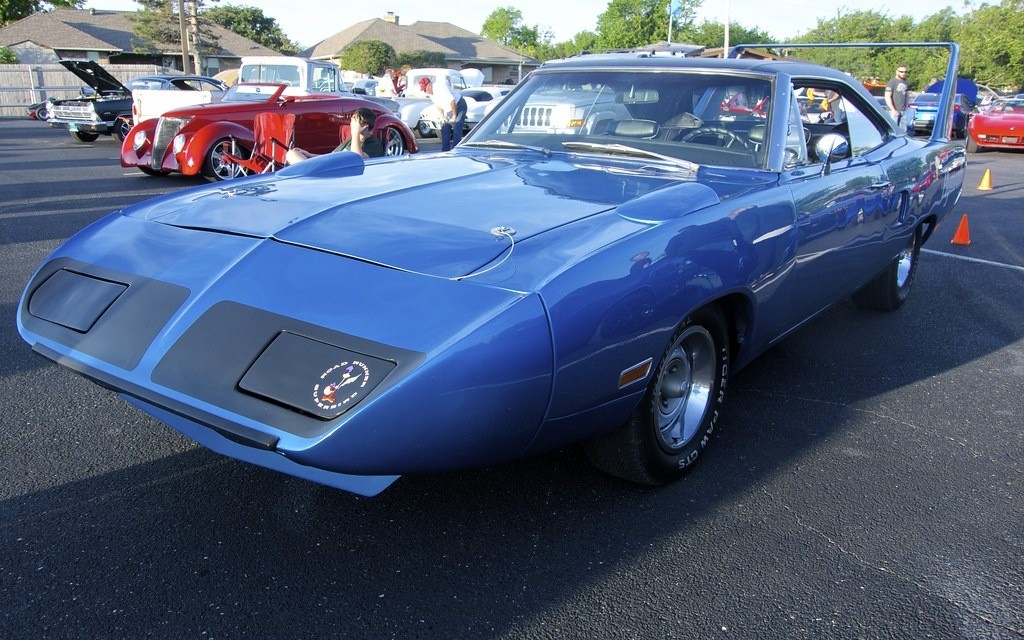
[898,70,908,74]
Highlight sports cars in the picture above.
[9,39,971,504]
[863,76,1024,153]
[46,58,228,144]
[116,80,419,183]
[25,101,50,122]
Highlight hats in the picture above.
[419,77,429,91]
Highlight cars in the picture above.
[332,57,518,137]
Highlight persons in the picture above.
[286,106,384,166]
[376,69,400,99]
[821,90,843,124]
[884,65,911,132]
[420,78,467,153]
[393,63,413,98]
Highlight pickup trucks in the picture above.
[126,55,402,126]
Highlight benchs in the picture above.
[655,120,851,159]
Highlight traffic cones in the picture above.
[977,169,993,191]
[951,213,973,245]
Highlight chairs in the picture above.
[338,125,390,157]
[600,120,660,143]
[748,125,811,162]
[222,111,296,179]
